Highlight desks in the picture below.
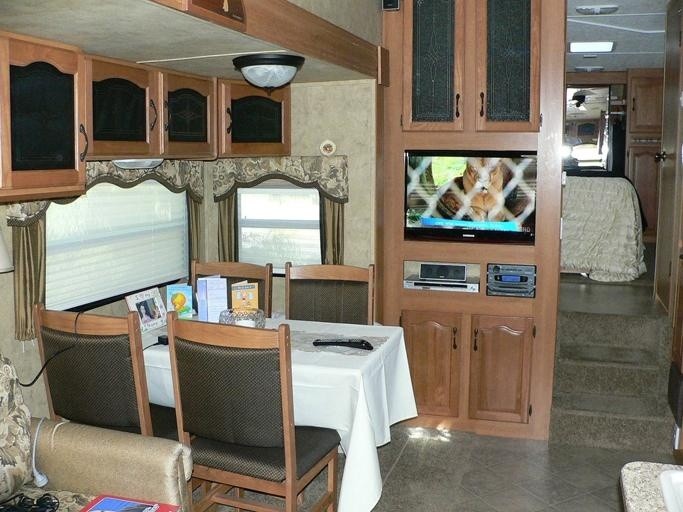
[619,461,683,512]
[102,309,418,512]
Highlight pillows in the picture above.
[0,351,35,503]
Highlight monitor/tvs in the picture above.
[404,150,537,245]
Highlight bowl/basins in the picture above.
[218,307,266,330]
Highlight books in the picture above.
[78,492,181,512]
[231,278,259,313]
[195,273,228,324]
[165,282,193,321]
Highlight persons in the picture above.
[138,305,153,324]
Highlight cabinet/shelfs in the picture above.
[626,68,663,243]
[85,52,219,159]
[1,27,88,202]
[217,78,291,159]
[380,0,567,439]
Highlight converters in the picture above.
[158,335,168,345]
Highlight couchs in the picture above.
[560,175,648,283]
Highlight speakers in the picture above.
[419,263,466,282]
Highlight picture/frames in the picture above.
[124,287,168,334]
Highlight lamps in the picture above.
[231,54,305,96]
[569,41,614,53]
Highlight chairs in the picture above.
[284,261,376,326]
[189,258,272,319]
[0,414,195,512]
[166,311,341,512]
[32,301,214,496]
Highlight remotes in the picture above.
[313,338,373,350]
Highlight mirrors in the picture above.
[562,82,627,178]
